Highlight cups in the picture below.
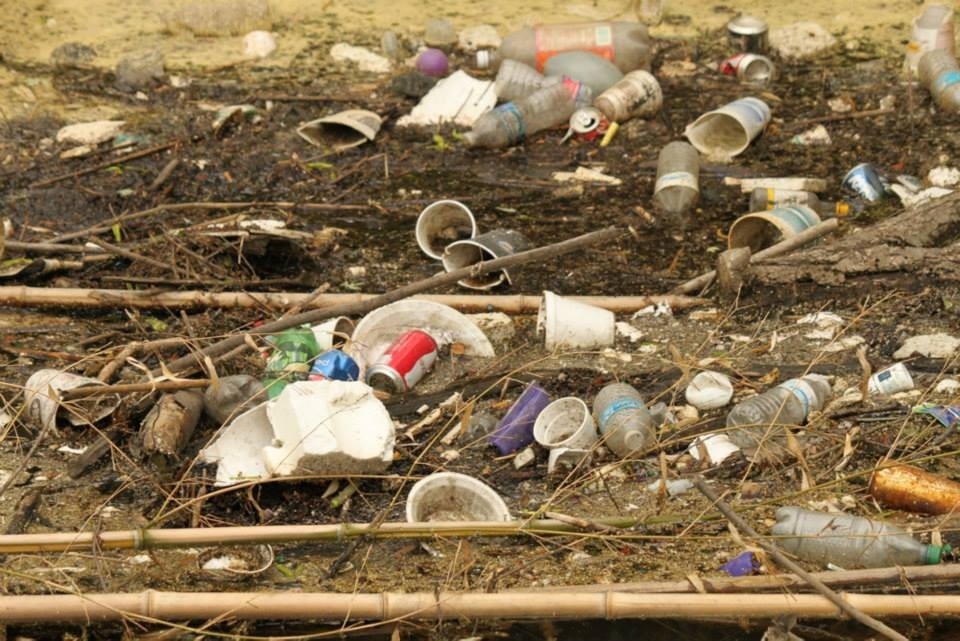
[413,69,822,458]
[24,368,121,437]
[297,110,383,150]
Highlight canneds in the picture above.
[718,16,774,89]
[307,349,359,382]
[568,106,610,142]
[841,162,886,204]
[865,455,960,516]
[365,329,440,395]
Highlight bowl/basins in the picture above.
[407,467,511,558]
[349,298,495,381]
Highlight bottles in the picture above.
[464,22,960,568]
[265,326,318,400]
